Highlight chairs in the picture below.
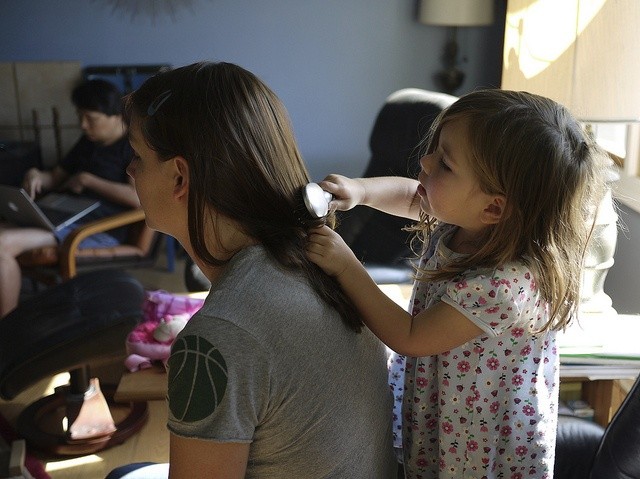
[335,86,460,266]
[58,64,170,283]
[556,378,639,479]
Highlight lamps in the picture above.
[417,0,496,93]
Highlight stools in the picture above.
[0,266,149,458]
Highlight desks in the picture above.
[115,288,639,428]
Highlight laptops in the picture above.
[1,186,101,230]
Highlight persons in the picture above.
[307,88,599,479]
[105,59,396,479]
[0,77,140,317]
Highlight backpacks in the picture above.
[124,290,206,374]
